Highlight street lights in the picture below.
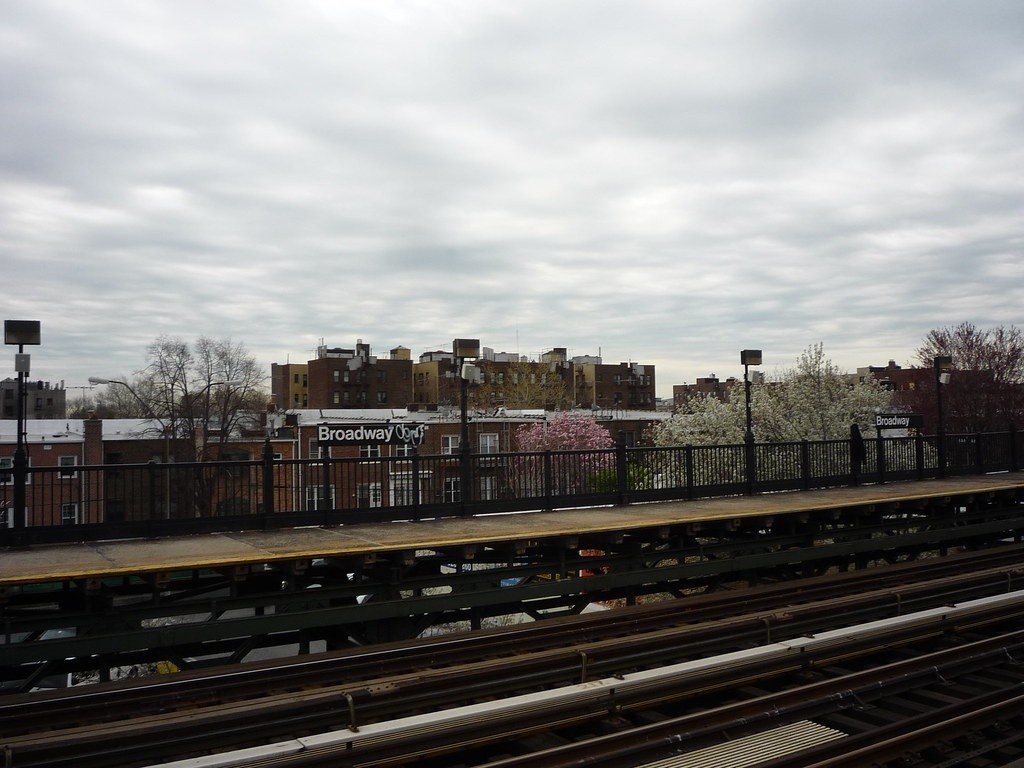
[454,338,481,519]
[4,319,42,547]
[934,355,953,479]
[87,377,244,535]
[740,349,762,496]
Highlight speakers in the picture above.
[461,364,475,379]
[748,370,759,383]
[940,373,951,384]
[15,354,30,372]
